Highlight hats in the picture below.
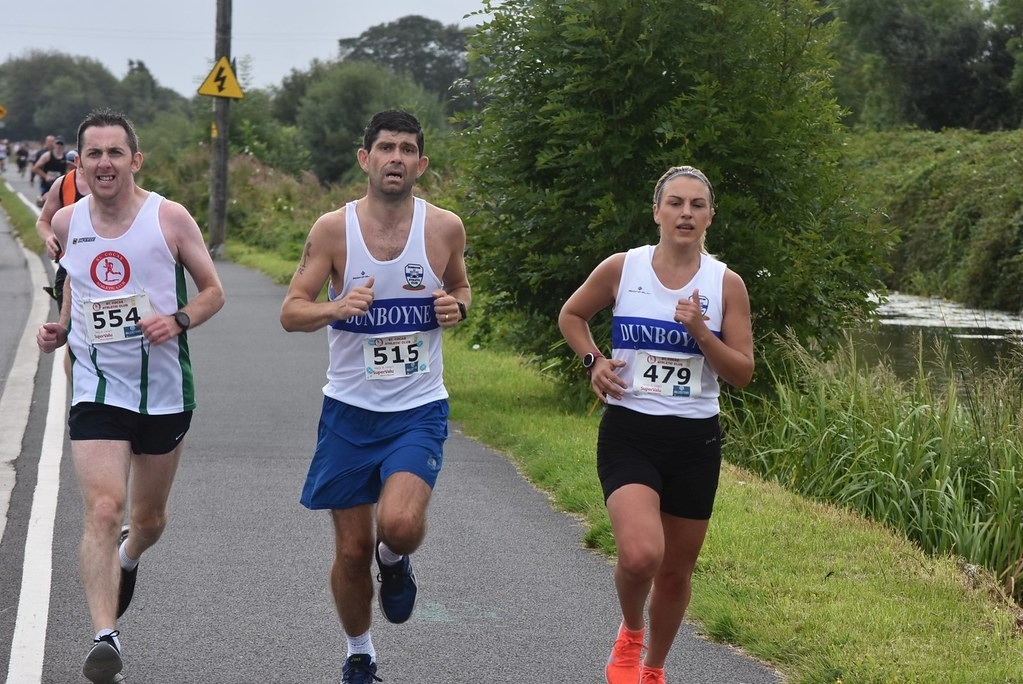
[55,136,64,145]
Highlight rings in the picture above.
[445,313,448,321]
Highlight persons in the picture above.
[0,138,12,171]
[14,141,37,179]
[33,135,79,196]
[36,168,92,382]
[558,166,755,684]
[36,106,226,684]
[280,110,472,684]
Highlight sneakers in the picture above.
[640,657,666,684]
[605,620,647,684]
[82,630,127,684]
[340,653,382,684]
[117,524,139,618]
[375,537,419,624]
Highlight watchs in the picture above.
[172,312,190,336]
[582,351,603,376]
[457,301,467,322]
